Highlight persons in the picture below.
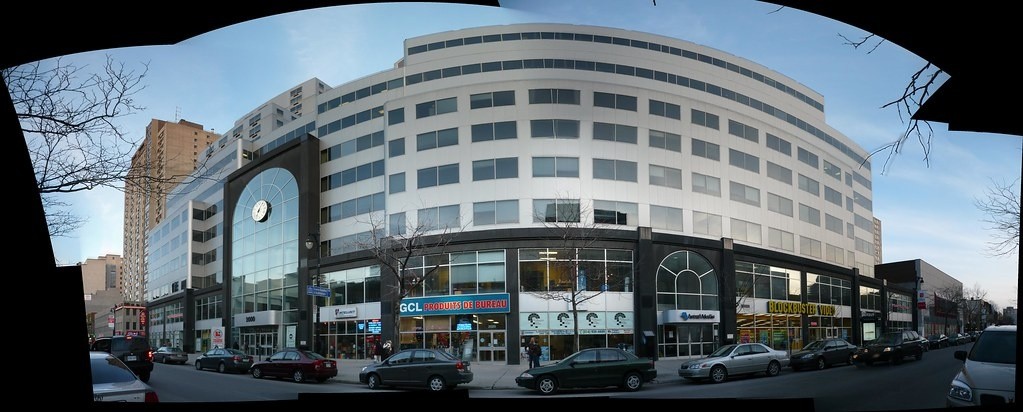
[374,339,394,364]
[162,342,166,347]
[233,342,239,350]
[167,341,172,347]
[525,337,540,368]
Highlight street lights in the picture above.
[303,232,322,352]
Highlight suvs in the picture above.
[90,335,154,384]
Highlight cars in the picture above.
[945,322,1017,408]
[928,330,984,350]
[851,330,929,369]
[90,350,159,403]
[678,342,791,384]
[152,347,188,365]
[789,338,857,371]
[515,348,657,395]
[359,349,474,391]
[250,349,338,384]
[195,348,254,375]
